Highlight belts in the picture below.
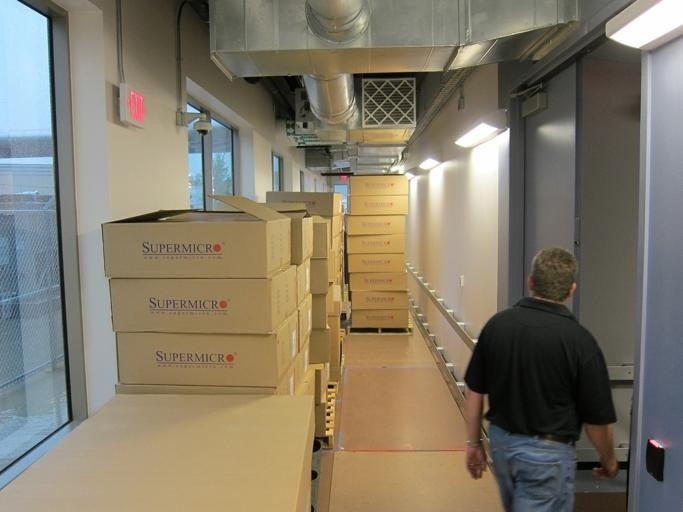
[508,431,576,448]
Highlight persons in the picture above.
[462,246,618,511]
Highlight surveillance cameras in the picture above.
[193,120,212,135]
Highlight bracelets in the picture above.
[463,437,481,448]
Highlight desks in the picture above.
[1,394,319,510]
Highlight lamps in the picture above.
[604,0,683,53]
